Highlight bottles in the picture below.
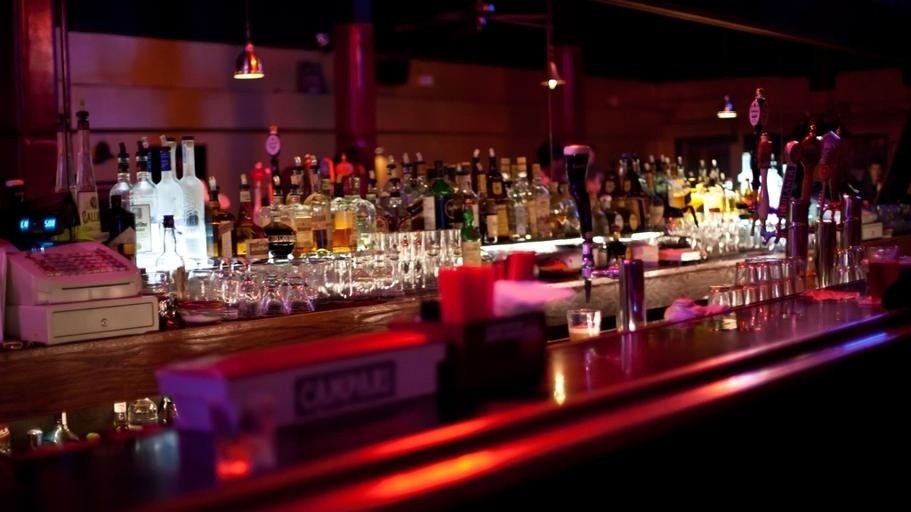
[103,133,209,298]
[595,149,733,235]
[210,149,576,262]
[51,112,75,245]
[73,110,104,242]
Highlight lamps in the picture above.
[717,94,738,118]
[540,1,565,90]
[231,17,265,80]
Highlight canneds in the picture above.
[707,259,794,308]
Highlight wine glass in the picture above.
[182,250,314,314]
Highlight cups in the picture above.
[708,255,806,309]
[826,246,867,287]
[357,227,463,292]
[567,308,602,341]
[276,249,407,301]
